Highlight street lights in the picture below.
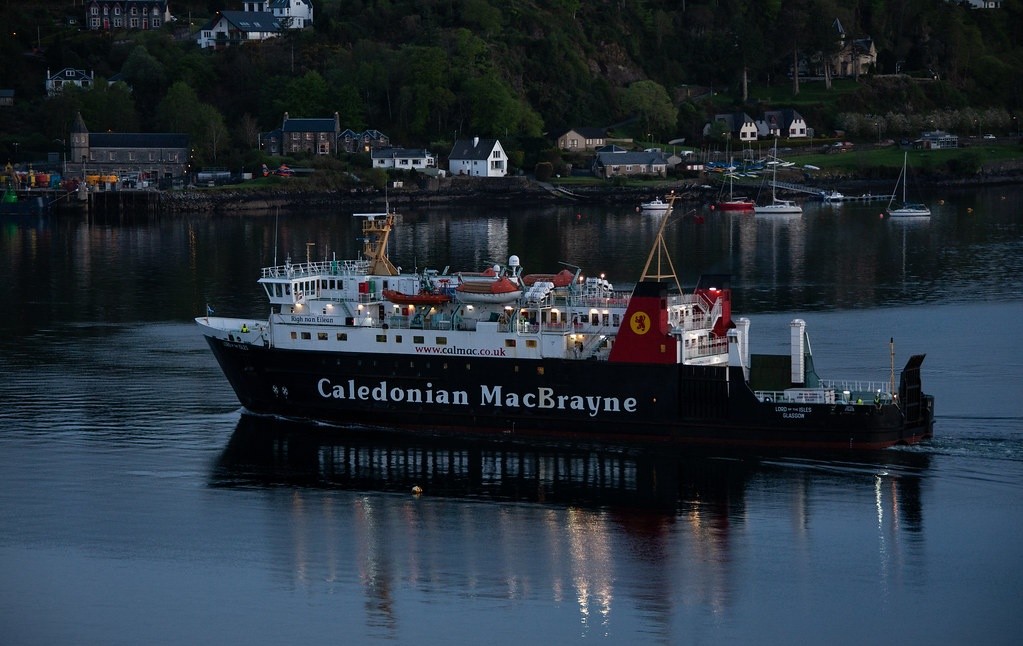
[648,133,655,149]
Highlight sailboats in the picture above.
[886,151,932,217]
[706,136,796,172]
[709,157,754,210]
[752,139,803,213]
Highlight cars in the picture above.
[984,133,996,139]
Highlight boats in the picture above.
[523,270,579,286]
[823,190,845,202]
[381,289,452,305]
[452,275,521,305]
[193,177,937,452]
[641,196,673,209]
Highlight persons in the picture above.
[856,396,863,405]
[874,395,881,404]
[498,309,510,324]
[241,323,248,333]
